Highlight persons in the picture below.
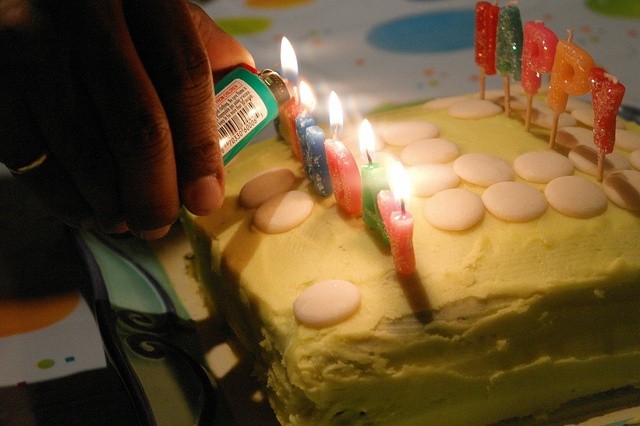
[1,0,256,241]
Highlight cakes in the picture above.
[177,80,640,426]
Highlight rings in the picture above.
[4,153,49,173]
